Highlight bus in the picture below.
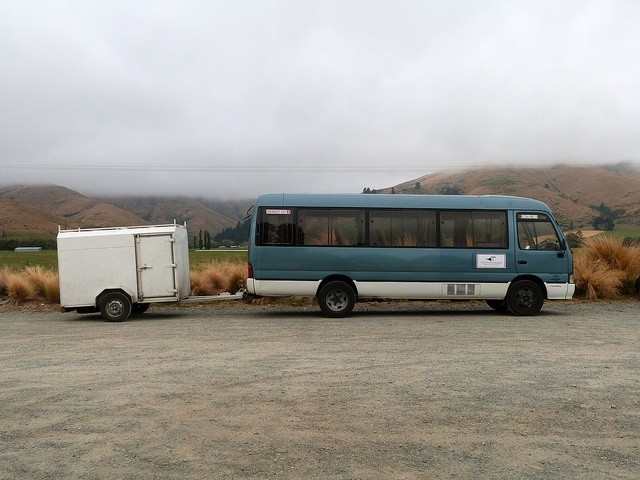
[244,195,577,314]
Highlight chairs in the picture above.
[334,226,349,245]
[376,227,390,247]
